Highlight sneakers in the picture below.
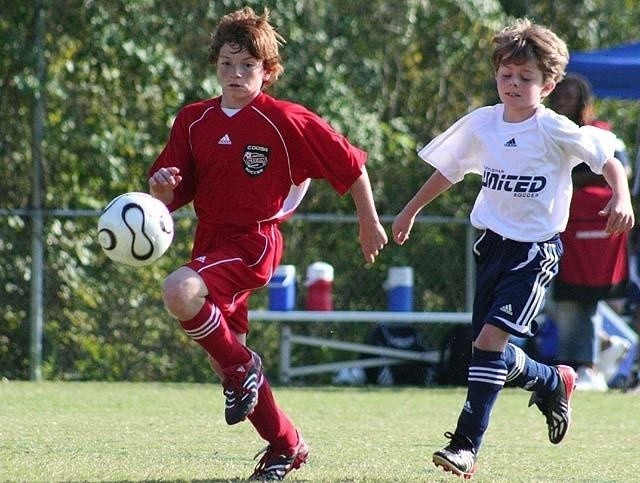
[597,337,629,381]
[573,366,607,391]
[432,431,479,478]
[529,365,577,443]
[222,345,263,425]
[244,430,310,482]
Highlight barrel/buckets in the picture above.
[265,265,297,311]
[380,266,417,312]
[301,262,332,311]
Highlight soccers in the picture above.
[98,191,175,268]
[332,368,367,386]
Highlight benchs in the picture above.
[246,310,487,385]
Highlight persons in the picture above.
[544,71,636,393]
[143,1,392,482]
[388,14,637,482]
